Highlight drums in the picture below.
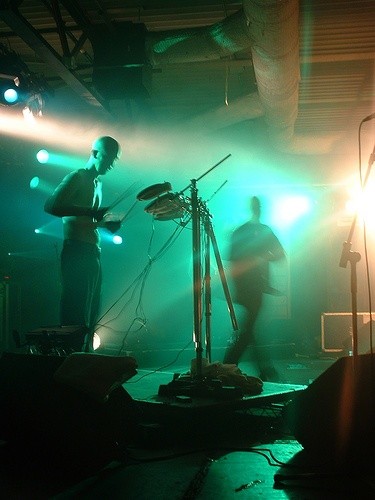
[136,182,171,201]
[145,193,184,221]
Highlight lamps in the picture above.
[0,83,22,107]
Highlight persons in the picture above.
[222,195,287,384]
[44,136,123,353]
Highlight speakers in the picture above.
[0,353,147,478]
[274,352,375,487]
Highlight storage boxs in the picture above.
[320,312,375,353]
